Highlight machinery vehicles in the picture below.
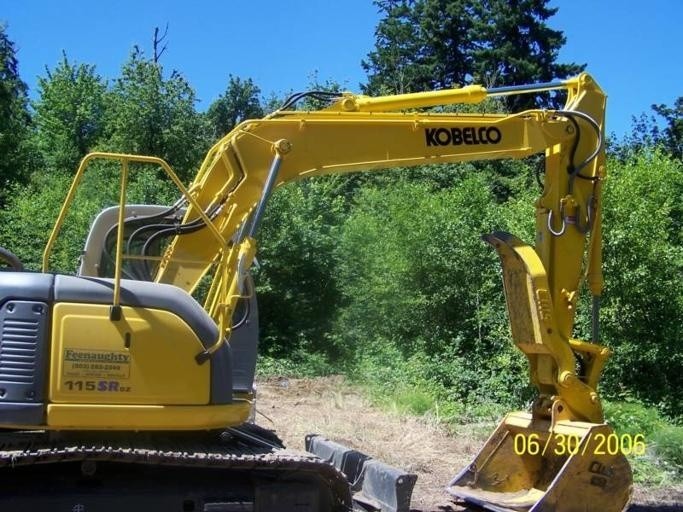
[0,71,634,511]
